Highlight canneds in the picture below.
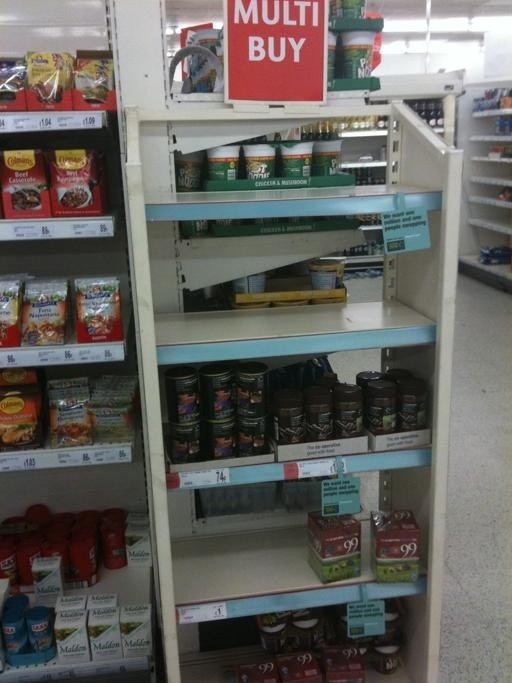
[163,360,427,465]
[0,504,127,655]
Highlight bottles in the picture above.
[301,99,445,141]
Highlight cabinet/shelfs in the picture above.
[458,105,512,280]
[123,97,464,683]
[1,108,158,683]
